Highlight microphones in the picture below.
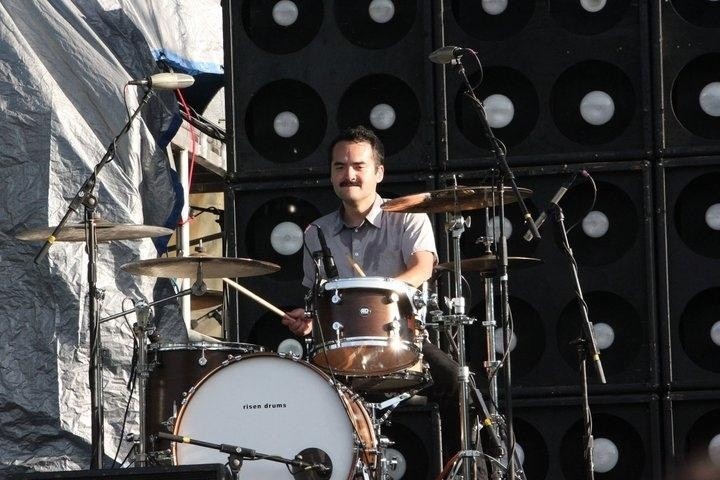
[151,71,196,90]
[134,302,161,345]
[191,304,221,328]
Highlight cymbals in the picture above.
[379,186,534,214]
[120,255,282,279]
[435,255,541,272]
[14,222,175,243]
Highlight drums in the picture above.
[339,351,433,402]
[303,276,425,377]
[161,348,380,480]
[139,341,272,468]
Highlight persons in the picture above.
[279,122,466,480]
